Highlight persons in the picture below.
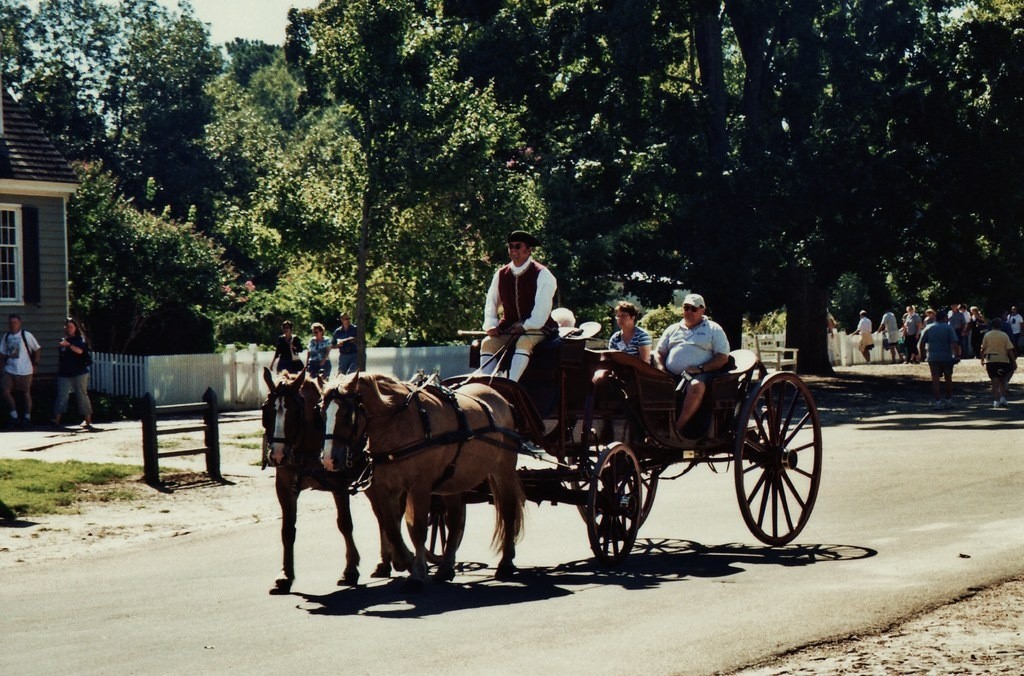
[478,230,557,383]
[653,294,730,430]
[851,302,1023,365]
[919,310,962,406]
[1,314,41,430]
[608,301,651,364]
[48,318,94,430]
[329,313,357,374]
[270,321,305,374]
[981,318,1016,409]
[305,322,332,376]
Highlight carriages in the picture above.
[261,309,825,598]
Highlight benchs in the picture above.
[584,348,676,411]
[755,334,799,371]
[470,326,586,369]
[700,349,756,410]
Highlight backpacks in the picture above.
[5,329,40,376]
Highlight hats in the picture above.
[507,229,543,248]
[683,293,706,309]
[935,311,948,321]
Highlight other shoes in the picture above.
[944,400,951,408]
[993,401,1000,408]
[889,359,920,364]
[934,400,942,410]
[8,415,20,429]
[49,418,59,426]
[999,398,1010,407]
[20,418,33,428]
[83,425,92,428]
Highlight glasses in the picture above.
[615,315,631,320]
[283,327,290,329]
[509,243,523,250]
[346,318,351,320]
[684,305,698,313]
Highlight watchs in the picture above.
[697,365,704,373]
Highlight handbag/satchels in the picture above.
[290,334,309,368]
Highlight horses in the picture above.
[261,366,523,595]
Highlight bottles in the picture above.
[61,338,66,351]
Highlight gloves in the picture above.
[508,326,525,344]
[488,328,505,337]
